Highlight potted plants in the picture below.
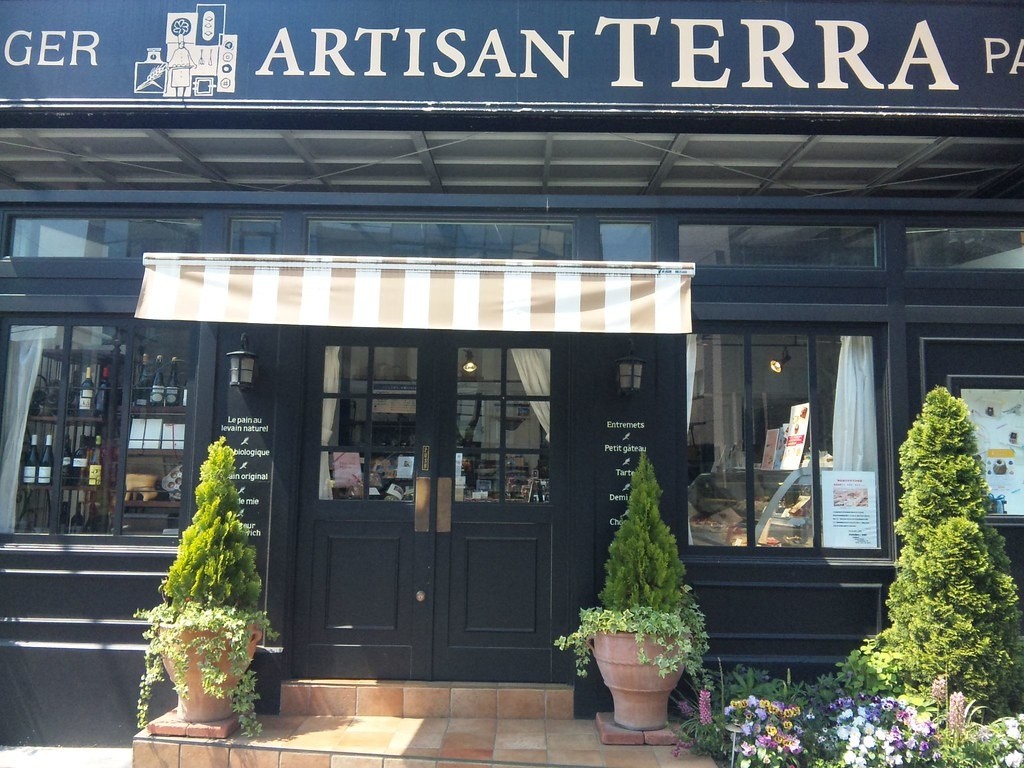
[554,450,715,731]
[133,436,284,738]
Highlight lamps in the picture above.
[614,339,647,396]
[770,346,791,373]
[225,333,259,391]
[463,350,477,373]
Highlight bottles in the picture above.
[164,356,181,405]
[39,435,54,485]
[22,435,39,483]
[93,368,110,414]
[134,353,152,406]
[89,436,103,487]
[128,491,135,514]
[74,435,89,483]
[84,503,100,533]
[59,502,70,534]
[62,438,74,486]
[150,354,166,407]
[136,493,144,514]
[79,366,95,416]
[70,501,81,534]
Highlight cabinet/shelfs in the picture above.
[688,470,822,547]
[14,349,186,534]
